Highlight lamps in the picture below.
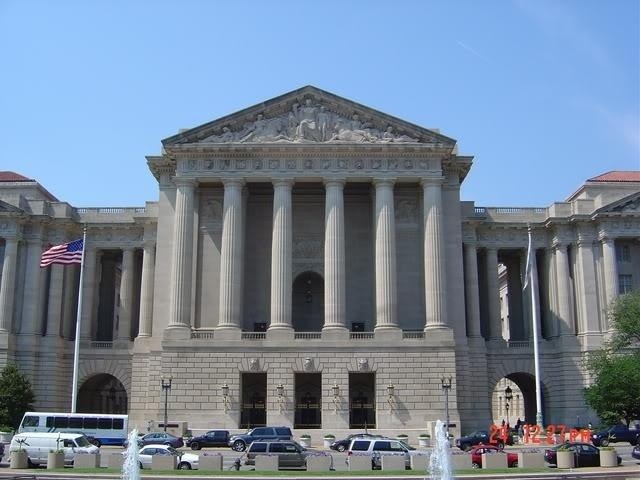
[220,380,397,413]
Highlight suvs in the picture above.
[244,439,327,470]
[228,427,295,452]
[342,438,432,472]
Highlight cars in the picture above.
[329,434,387,452]
[543,443,623,467]
[121,430,184,450]
[458,443,522,468]
[631,445,640,461]
[122,444,200,472]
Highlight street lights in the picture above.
[159,374,174,436]
[439,374,454,448]
[505,402,510,428]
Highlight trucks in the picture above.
[8,432,102,467]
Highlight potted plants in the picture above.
[556,447,617,469]
[397,434,455,448]
[182,433,193,446]
[0,424,14,444]
[299,434,335,450]
[9,449,64,469]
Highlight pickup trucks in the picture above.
[590,421,640,446]
[455,429,518,451]
[185,430,234,451]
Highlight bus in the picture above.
[18,411,129,449]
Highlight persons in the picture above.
[201,98,419,144]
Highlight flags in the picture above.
[40,237,83,268]
[523,239,533,289]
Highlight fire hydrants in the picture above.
[234,458,243,471]
